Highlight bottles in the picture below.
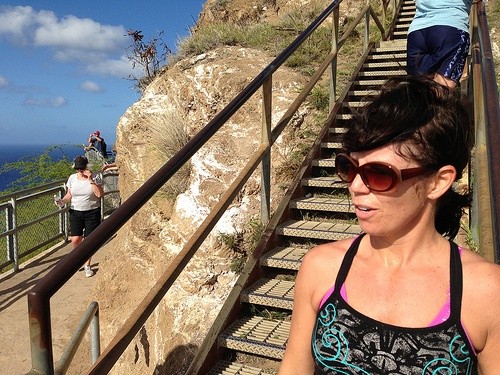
[53,195,64,209]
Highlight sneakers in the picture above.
[84,264,94,277]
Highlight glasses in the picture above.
[334,152,426,192]
[76,168,85,170]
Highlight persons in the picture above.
[55,156,102,278]
[84,130,106,158]
[280,81,500,375]
[407,0,472,87]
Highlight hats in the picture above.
[74,155,88,169]
[93,130,100,135]
